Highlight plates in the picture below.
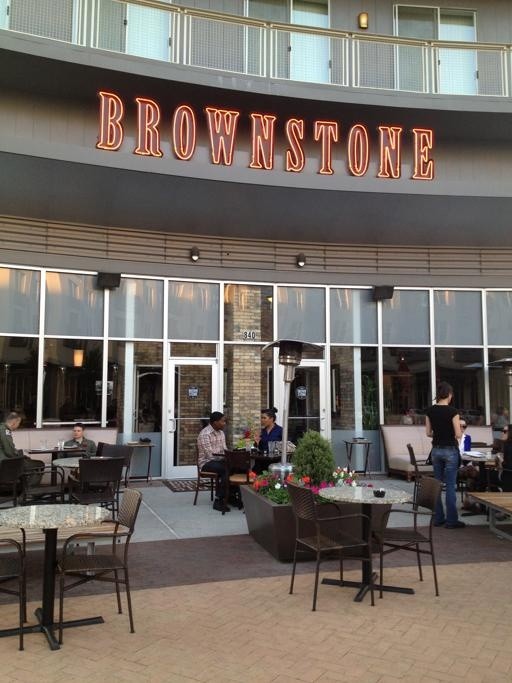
[127,440,140,445]
[464,449,485,458]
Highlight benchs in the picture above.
[379,424,494,483]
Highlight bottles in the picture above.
[245,438,253,453]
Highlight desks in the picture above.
[124,441,158,486]
[469,492,512,542]
[0,503,108,652]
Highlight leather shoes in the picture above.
[214,499,229,511]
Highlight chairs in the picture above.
[486,467,512,520]
[0,524,32,651]
[194,439,217,504]
[222,450,257,515]
[285,479,374,611]
[0,439,134,518]
[51,488,141,642]
[406,444,432,482]
[373,475,442,598]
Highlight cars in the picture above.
[363,398,486,427]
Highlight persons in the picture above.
[427,381,464,528]
[197,411,242,512]
[252,406,283,475]
[64,422,97,455]
[456,416,512,522]
[1,409,45,504]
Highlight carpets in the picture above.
[161,478,218,491]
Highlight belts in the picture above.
[433,444,458,450]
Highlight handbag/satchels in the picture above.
[266,440,295,453]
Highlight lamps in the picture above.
[188,246,202,263]
[296,252,307,268]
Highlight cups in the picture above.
[57,438,65,450]
[39,438,48,450]
[354,486,362,499]
[268,440,276,454]
[372,487,386,498]
[486,450,491,459]
